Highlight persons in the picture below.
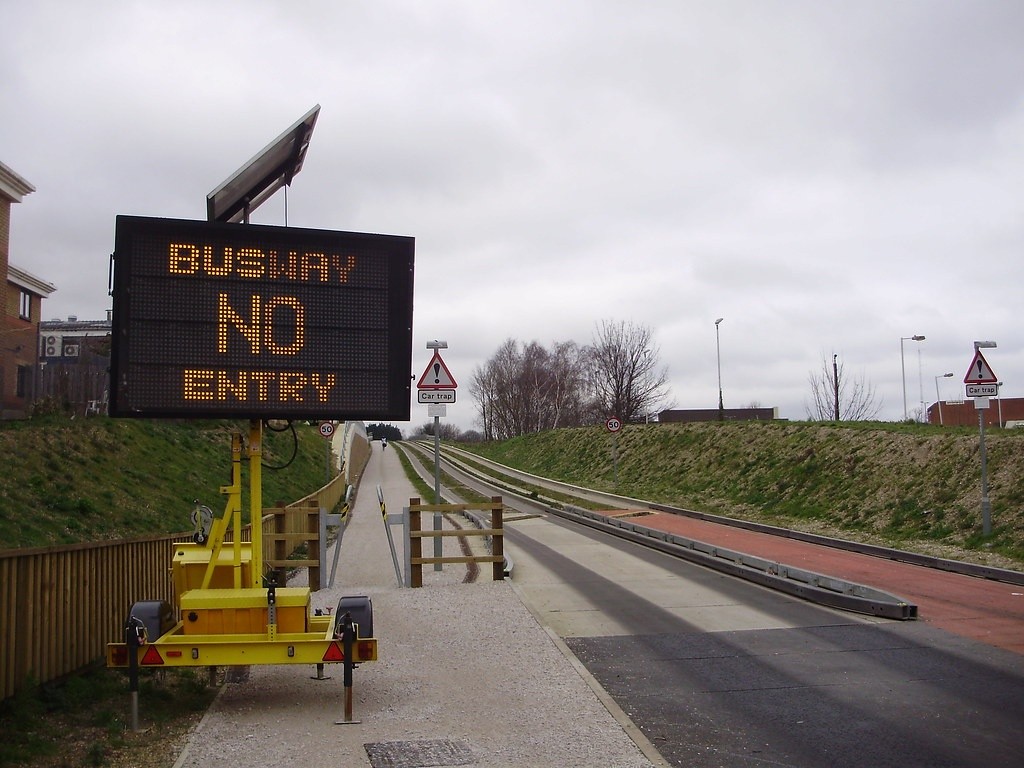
[382,436,387,452]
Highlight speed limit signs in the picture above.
[606,417,621,432]
[320,422,334,437]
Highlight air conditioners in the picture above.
[64,345,79,356]
[45,335,63,345]
[46,345,62,356]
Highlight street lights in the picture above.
[997,382,1006,428]
[900,336,926,417]
[643,348,651,424]
[833,353,840,421]
[715,318,727,421]
[935,373,953,427]
